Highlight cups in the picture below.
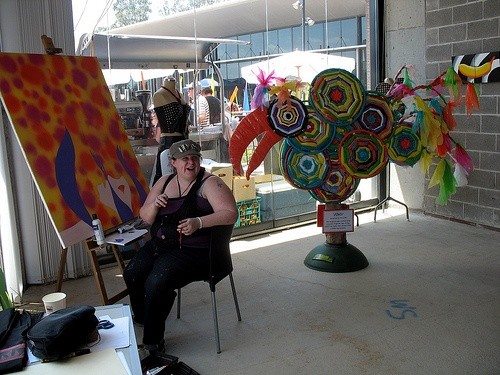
[42,293,67,315]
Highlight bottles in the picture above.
[93,214,105,245]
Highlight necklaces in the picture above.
[176,174,198,197]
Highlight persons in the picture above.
[202,88,221,124]
[152,77,191,187]
[184,81,210,125]
[122,140,239,354]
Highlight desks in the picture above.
[55,217,151,307]
[0,304,143,375]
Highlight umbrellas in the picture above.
[240,47,356,86]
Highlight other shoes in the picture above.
[137,333,165,356]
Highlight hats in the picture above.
[170,139,201,158]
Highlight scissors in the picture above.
[97,319,115,329]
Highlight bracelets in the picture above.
[197,217,203,229]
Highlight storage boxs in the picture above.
[232,196,262,228]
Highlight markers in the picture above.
[41,348,91,363]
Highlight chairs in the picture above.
[176,207,243,354]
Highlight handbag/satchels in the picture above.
[20,305,99,359]
